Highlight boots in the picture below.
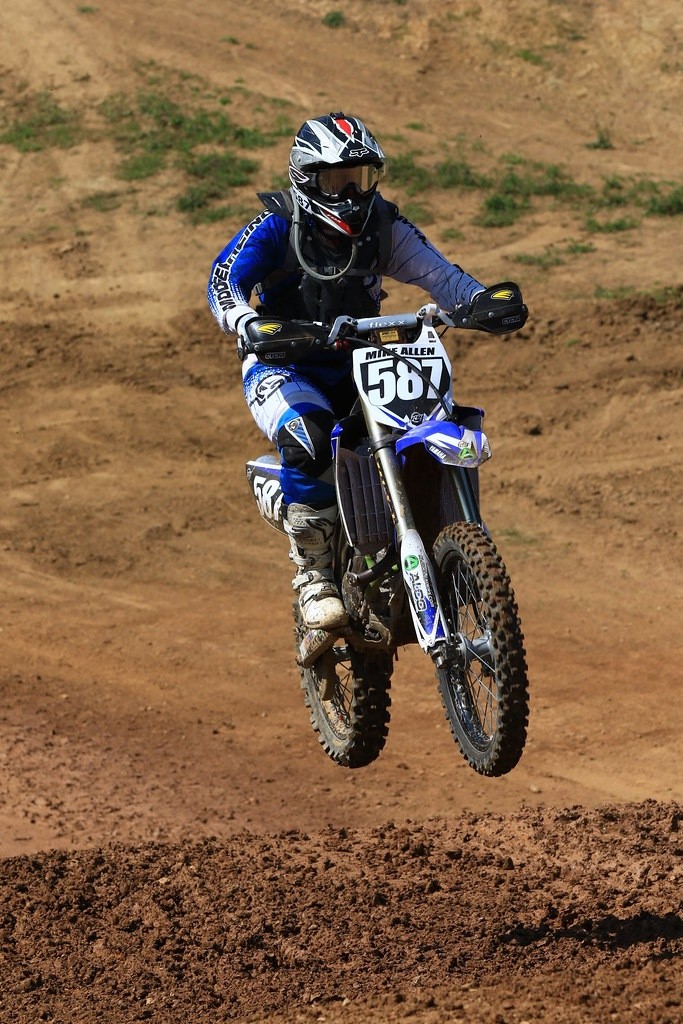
[282,503,349,630]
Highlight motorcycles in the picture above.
[208,112,530,778]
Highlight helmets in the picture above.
[286,111,387,243]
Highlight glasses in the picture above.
[314,163,381,196]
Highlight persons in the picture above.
[205,114,529,629]
